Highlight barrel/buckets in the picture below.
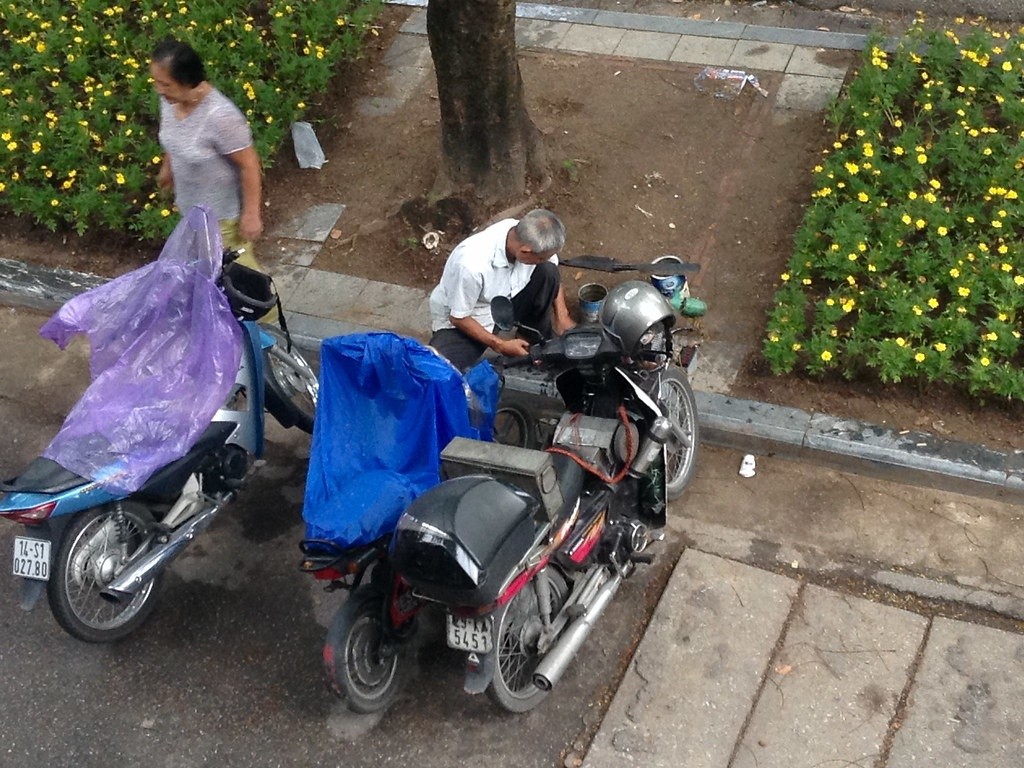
[578,283,608,324]
[647,255,692,303]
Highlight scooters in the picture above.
[297,334,537,715]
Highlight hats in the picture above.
[598,279,676,356]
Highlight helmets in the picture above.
[224,264,278,323]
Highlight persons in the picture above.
[150,40,284,331]
[423,208,579,374]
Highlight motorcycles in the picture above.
[1,205,321,646]
[384,282,702,715]
